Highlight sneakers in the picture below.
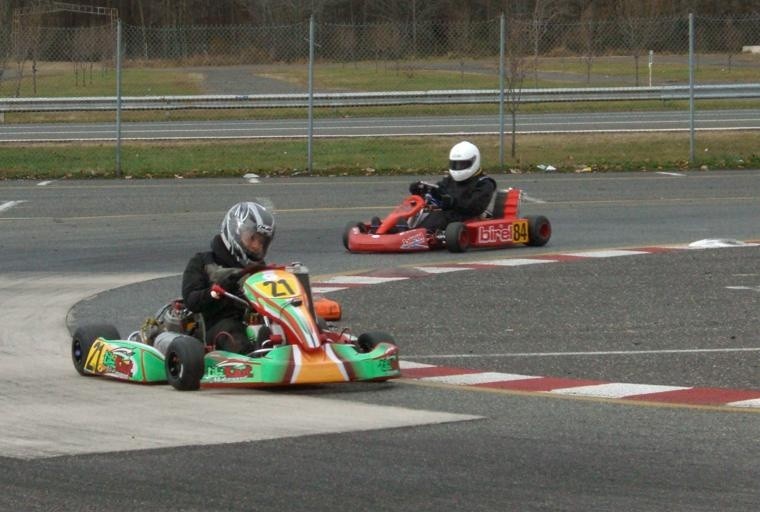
[396,218,409,233]
[250,325,273,358]
[370,216,381,233]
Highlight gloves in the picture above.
[409,182,428,195]
[215,270,245,301]
[438,193,455,212]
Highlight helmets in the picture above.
[219,199,276,276]
[447,140,481,182]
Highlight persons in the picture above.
[367,140,498,235]
[181,200,330,356]
